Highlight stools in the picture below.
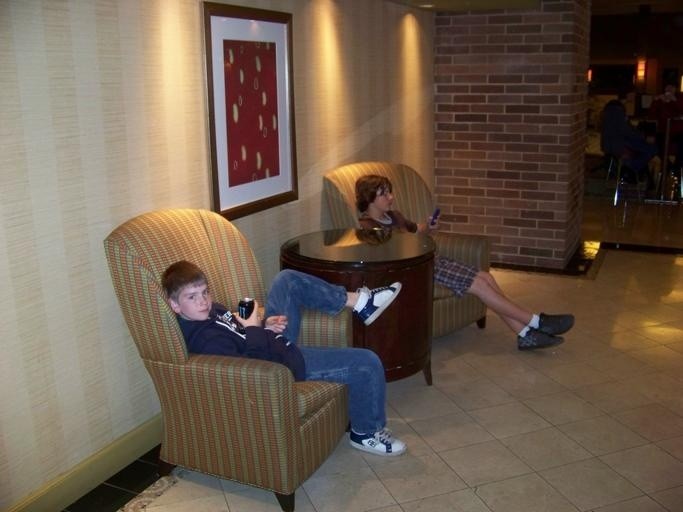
[606,141,641,207]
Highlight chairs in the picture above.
[100,207,356,512]
[321,162,492,335]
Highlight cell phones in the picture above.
[430,208,440,225]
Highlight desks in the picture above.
[277,228,440,385]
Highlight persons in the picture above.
[600,85,683,201]
[354,175,574,350]
[162,261,407,458]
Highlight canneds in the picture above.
[238,297,254,333]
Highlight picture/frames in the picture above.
[201,0,299,224]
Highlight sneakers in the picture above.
[348,428,407,458]
[531,312,575,336]
[517,326,565,351]
[352,282,403,325]
